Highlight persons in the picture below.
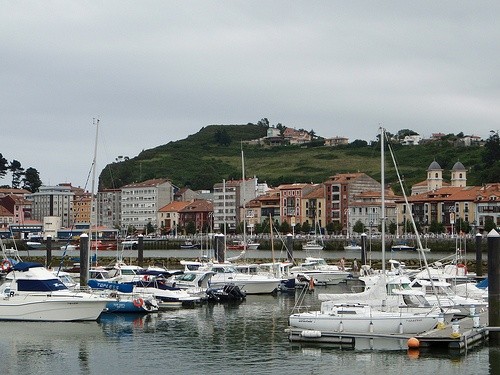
[340,257,345,271]
[353,258,358,272]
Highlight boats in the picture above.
[179,239,198,252]
[344,241,361,250]
[0,119,490,353]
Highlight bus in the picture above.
[8,225,44,235]
[56,230,120,240]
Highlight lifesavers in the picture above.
[134,319,142,326]
[458,264,467,275]
[134,299,143,306]
[1,260,11,271]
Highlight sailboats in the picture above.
[390,214,416,251]
[301,216,326,251]
